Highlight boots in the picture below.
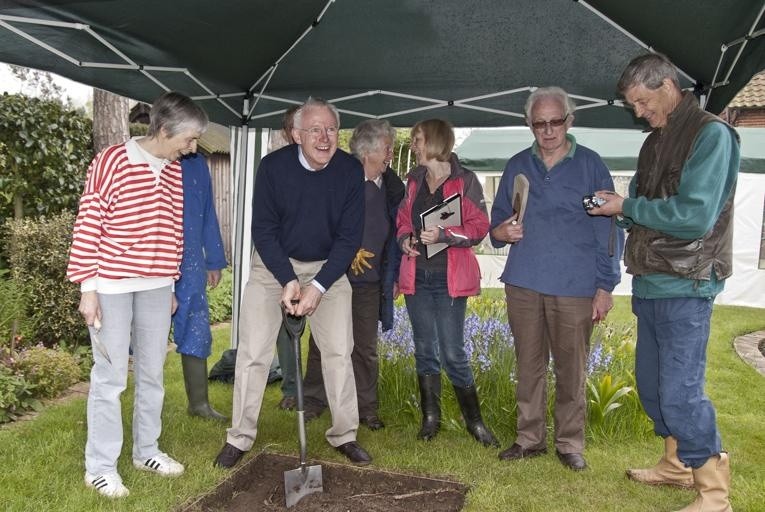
[672,451,733,512]
[182,353,229,423]
[625,436,695,488]
[452,384,501,449]
[417,373,441,440]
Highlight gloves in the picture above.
[351,249,375,276]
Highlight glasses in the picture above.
[532,113,569,129]
[296,126,338,137]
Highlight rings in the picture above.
[422,241,425,245]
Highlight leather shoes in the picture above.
[556,450,586,471]
[498,443,547,460]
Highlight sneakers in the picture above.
[359,415,385,430]
[84,470,130,498]
[335,442,373,467]
[304,403,322,422]
[213,443,244,470]
[133,452,185,477]
[279,396,297,409]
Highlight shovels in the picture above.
[280,299,324,510]
[92,319,112,364]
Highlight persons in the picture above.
[212,98,374,468]
[66,93,209,500]
[275,108,305,411]
[587,56,743,512]
[394,119,503,448]
[487,87,626,470]
[303,119,407,430]
[172,150,232,424]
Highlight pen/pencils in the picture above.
[408,233,414,261]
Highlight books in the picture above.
[420,193,464,260]
[511,174,529,225]
